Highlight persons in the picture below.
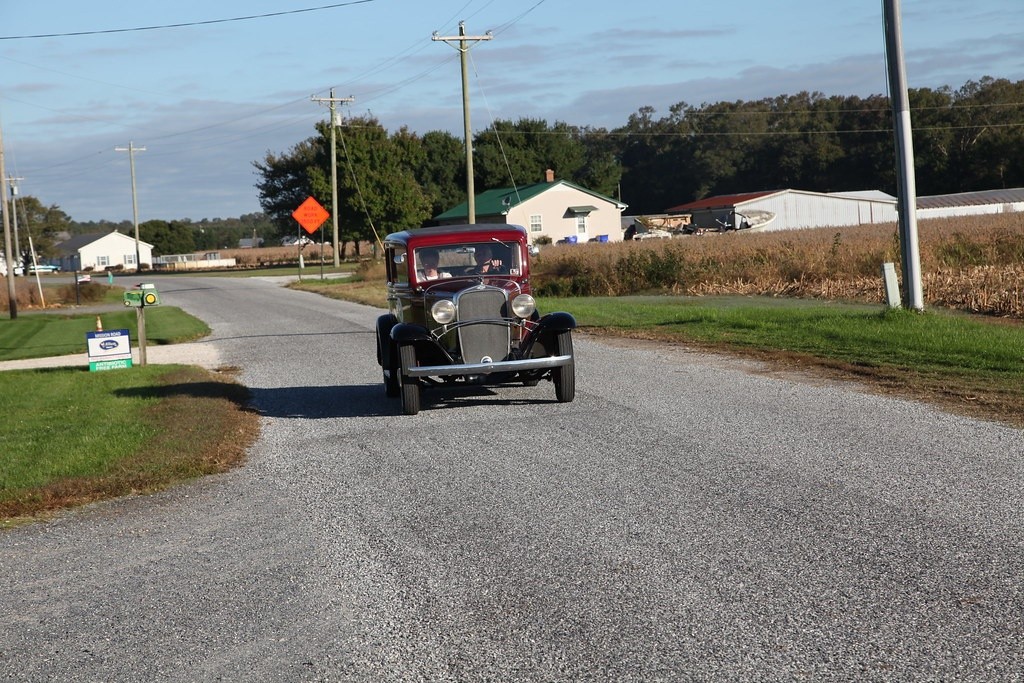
[466,245,509,275]
[417,247,452,283]
[105,267,113,285]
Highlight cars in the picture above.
[22,261,62,276]
[376,225,575,415]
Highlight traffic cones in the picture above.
[96,316,102,332]
